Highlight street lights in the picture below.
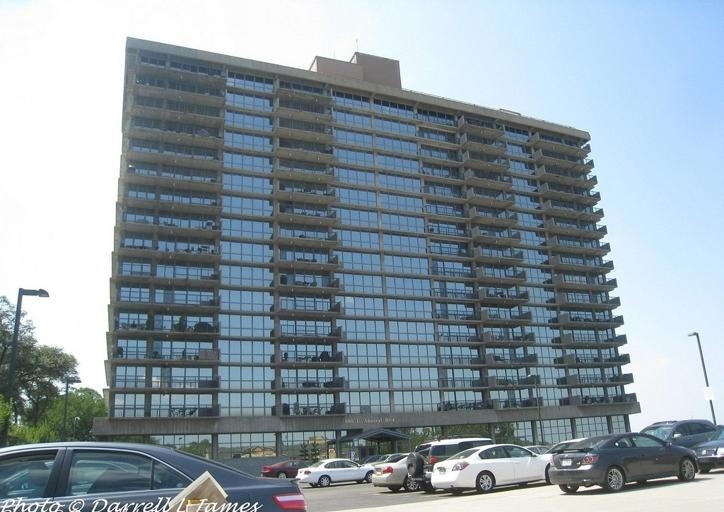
[3,288,50,446]
[61,375,81,442]
[73,416,81,440]
[688,332,717,425]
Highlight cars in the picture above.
[0,441,307,512]
